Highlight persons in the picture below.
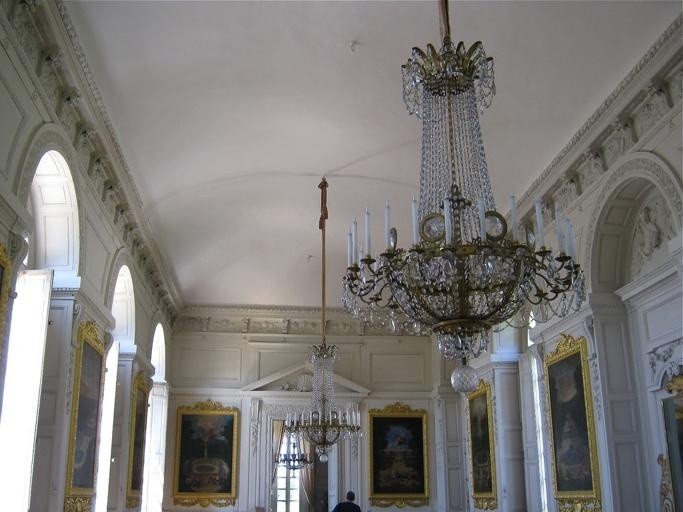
[334,490,361,511]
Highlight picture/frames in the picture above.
[61,319,106,512]
[170,399,240,507]
[656,365,683,512]
[367,402,431,510]
[467,382,499,512]
[0,243,14,343]
[544,331,604,512]
[125,370,154,509]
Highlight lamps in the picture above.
[284,173,361,464]
[340,1,587,396]
[272,429,314,479]
